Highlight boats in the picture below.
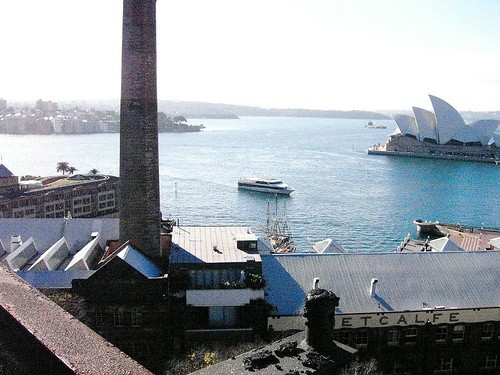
[365,121,386,128]
[412,220,439,232]
[435,223,500,237]
[237,176,295,195]
